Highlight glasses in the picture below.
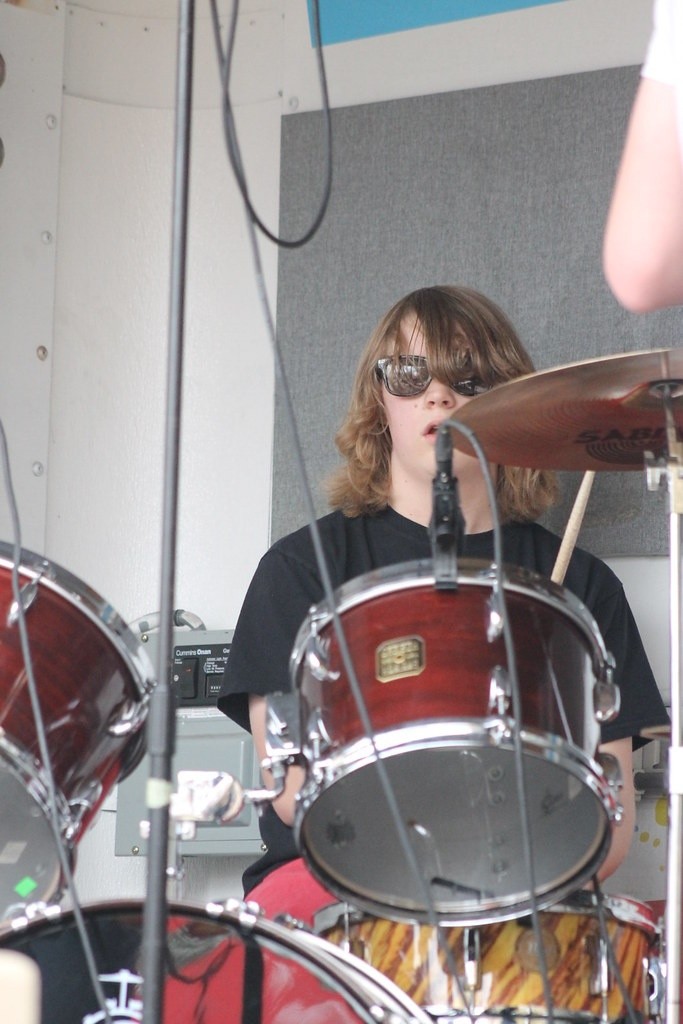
[375,355,492,396]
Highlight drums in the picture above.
[295,886,666,1024]
[0,535,154,927]
[0,894,434,1024]
[290,553,619,930]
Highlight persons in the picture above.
[603,0,683,313]
[215,288,672,1024]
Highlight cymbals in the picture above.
[437,342,682,471]
